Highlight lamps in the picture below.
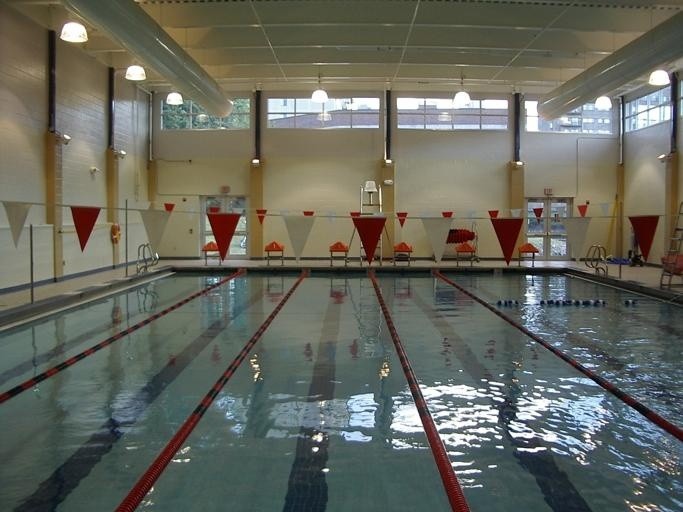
[437,112,452,122]
[311,74,329,104]
[318,112,332,121]
[648,68,671,86]
[594,95,613,111]
[60,15,184,107]
[196,113,208,123]
[453,73,471,106]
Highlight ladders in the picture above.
[360,184,382,267]
[659,202,682,290]
